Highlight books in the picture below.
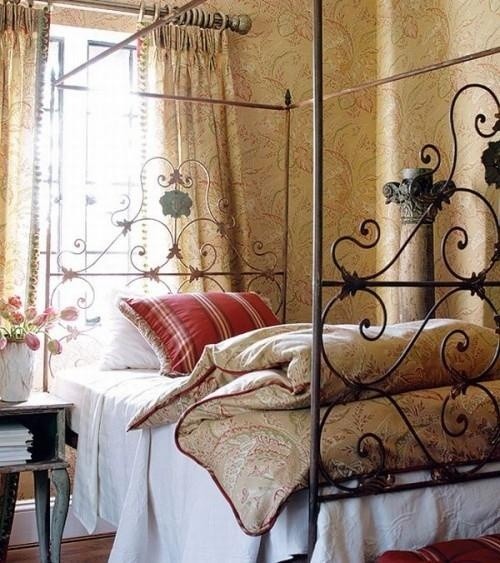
[0,421,34,467]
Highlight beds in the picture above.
[48,85,500,562]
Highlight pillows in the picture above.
[93,290,283,376]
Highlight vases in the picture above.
[0,341,34,402]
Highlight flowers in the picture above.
[0,295,78,378]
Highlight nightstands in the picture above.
[1,392,75,562]
